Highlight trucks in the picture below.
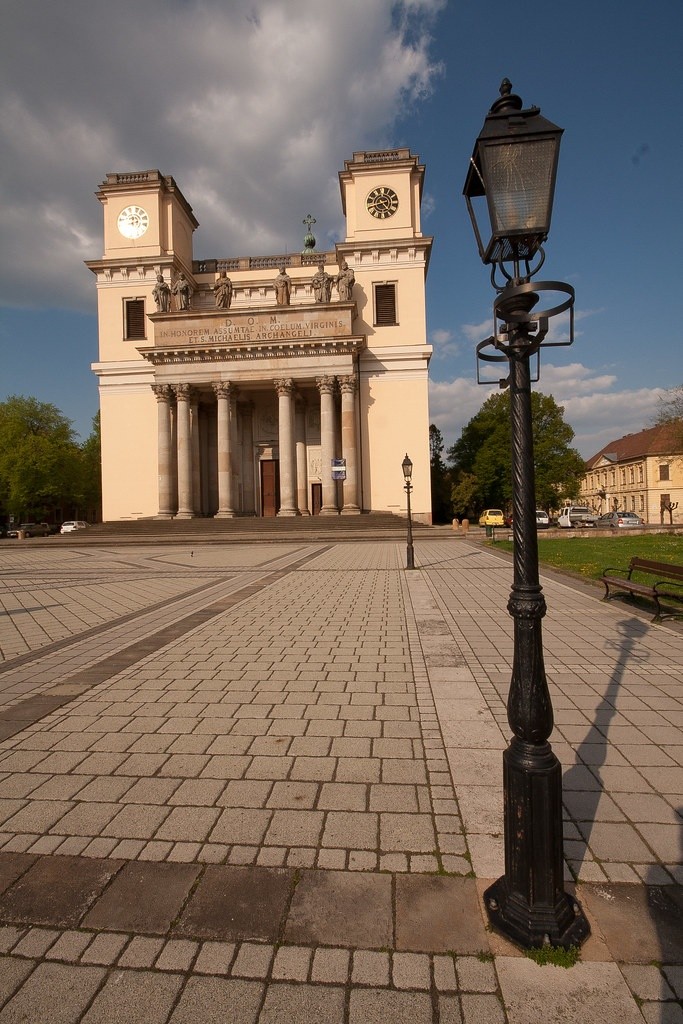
[556,506,602,529]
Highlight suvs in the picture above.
[535,511,550,530]
[7,522,50,538]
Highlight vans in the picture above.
[478,509,505,528]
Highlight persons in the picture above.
[310,264,334,305]
[152,275,171,313]
[273,266,292,306]
[171,272,193,311]
[213,269,232,310]
[334,261,355,302]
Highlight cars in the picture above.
[594,511,645,528]
[506,513,513,530]
[60,521,90,534]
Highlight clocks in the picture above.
[118,206,149,238]
[366,187,399,219]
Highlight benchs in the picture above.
[599,556,683,623]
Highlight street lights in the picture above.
[403,453,417,573]
[461,78,592,959]
